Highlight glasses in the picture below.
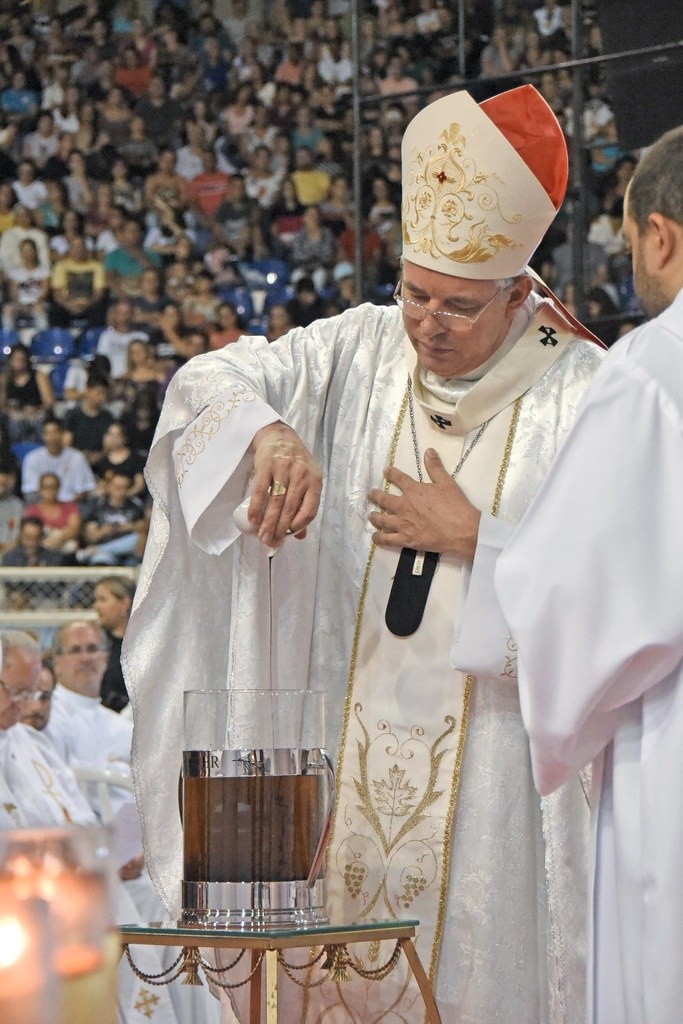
[394,275,515,331]
[40,691,57,705]
[0,680,43,704]
[62,642,112,656]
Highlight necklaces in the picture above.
[404,370,489,575]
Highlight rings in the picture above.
[270,480,287,496]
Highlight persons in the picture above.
[178,183,628,1021]
[90,574,142,714]
[494,125,681,1023]
[21,657,221,1024]
[2,0,641,617]
[47,621,129,799]
[0,628,172,1024]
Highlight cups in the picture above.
[178,684,340,930]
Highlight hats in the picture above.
[401,82,569,279]
